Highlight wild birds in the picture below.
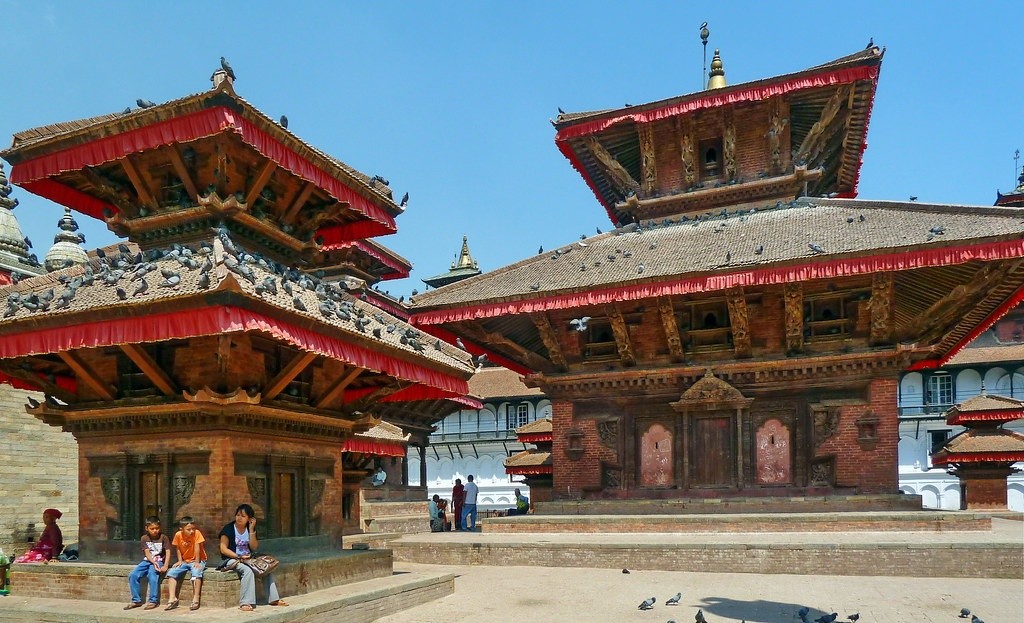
[638,597,657,611]
[477,353,487,363]
[455,338,468,351]
[866,37,875,48]
[557,106,565,114]
[565,315,593,333]
[959,607,985,623]
[666,592,682,605]
[624,103,633,107]
[909,194,918,201]
[15,197,19,205]
[694,608,708,623]
[798,605,860,623]
[118,55,409,210]
[926,222,945,242]
[527,157,866,294]
[0,235,444,359]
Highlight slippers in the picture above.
[164,599,179,610]
[190,601,199,609]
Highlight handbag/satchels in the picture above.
[244,553,279,576]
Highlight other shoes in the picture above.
[241,605,253,611]
[269,600,289,606]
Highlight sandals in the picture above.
[144,603,159,610]
[123,602,142,610]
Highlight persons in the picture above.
[642,140,655,179]
[763,114,787,166]
[370,465,387,487]
[508,488,530,516]
[728,292,746,339]
[785,287,803,337]
[122,517,207,611]
[658,301,683,354]
[11,509,66,564]
[681,133,693,180]
[219,505,290,612]
[608,311,632,357]
[866,273,890,331]
[723,121,736,173]
[537,318,563,361]
[428,475,479,533]
